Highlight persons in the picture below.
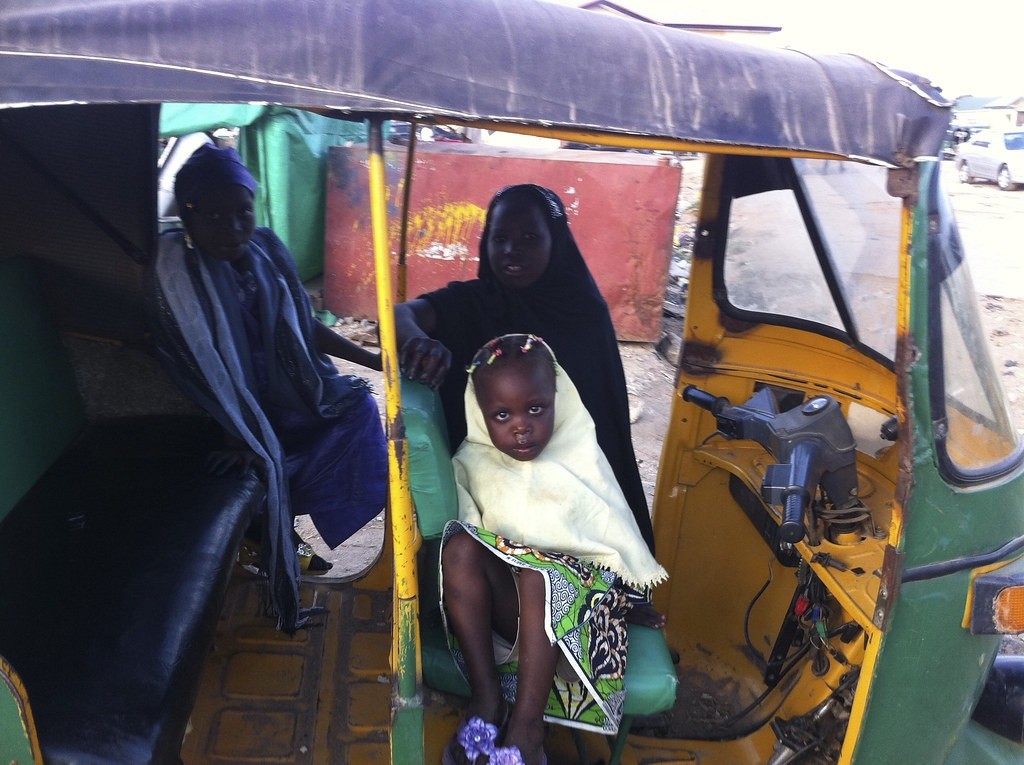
[137,141,389,578]
[437,332,670,765]
[373,182,668,630]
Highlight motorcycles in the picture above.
[2,0,1023,763]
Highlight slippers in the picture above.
[480,746,548,765]
[237,531,332,576]
[440,694,511,765]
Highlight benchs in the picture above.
[396,374,680,765]
[0,254,268,764]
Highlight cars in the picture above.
[952,127,1023,192]
